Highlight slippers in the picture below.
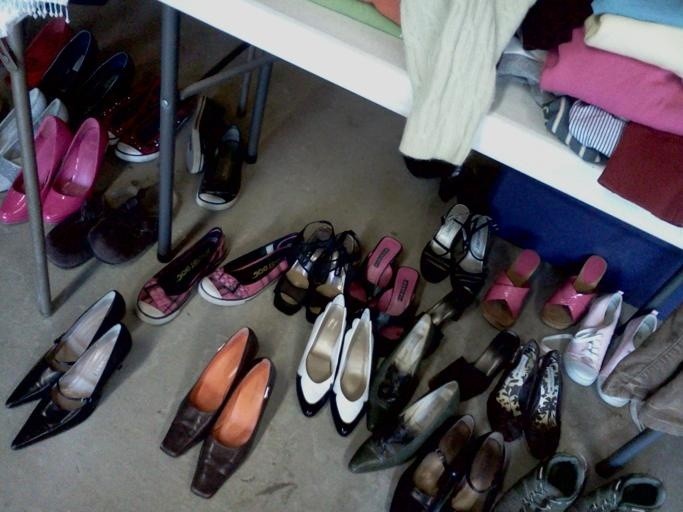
[542,255,607,332]
[42,182,139,269]
[88,181,184,265]
[398,285,475,362]
[428,329,520,400]
[373,266,421,357]
[344,236,404,325]
[483,250,540,329]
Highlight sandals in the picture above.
[448,212,502,302]
[305,230,362,323]
[273,219,336,317]
[421,203,469,285]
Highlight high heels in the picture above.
[5,290,127,408]
[9,324,133,451]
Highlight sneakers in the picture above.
[596,309,660,408]
[540,289,623,388]
[565,474,667,512]
[98,78,245,213]
[492,454,586,511]
[198,233,304,306]
[136,227,228,325]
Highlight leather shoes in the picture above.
[190,357,276,499]
[161,327,258,460]
[389,415,475,512]
[524,349,563,459]
[367,314,431,430]
[439,432,506,512]
[0,18,135,226]
[295,294,346,415]
[486,338,540,443]
[348,379,460,474]
[331,308,375,436]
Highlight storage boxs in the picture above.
[484,166,681,319]
[157,0,682,477]
